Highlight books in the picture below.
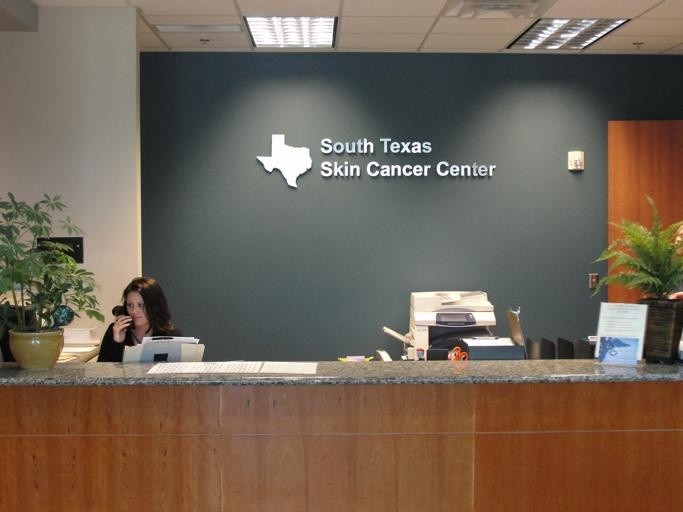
[593,301,648,367]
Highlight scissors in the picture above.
[448,347,468,360]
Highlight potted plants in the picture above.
[591,193,683,361]
[1,190,105,373]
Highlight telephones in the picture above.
[112,306,136,329]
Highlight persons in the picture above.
[666,224,683,301]
[94,276,183,362]
